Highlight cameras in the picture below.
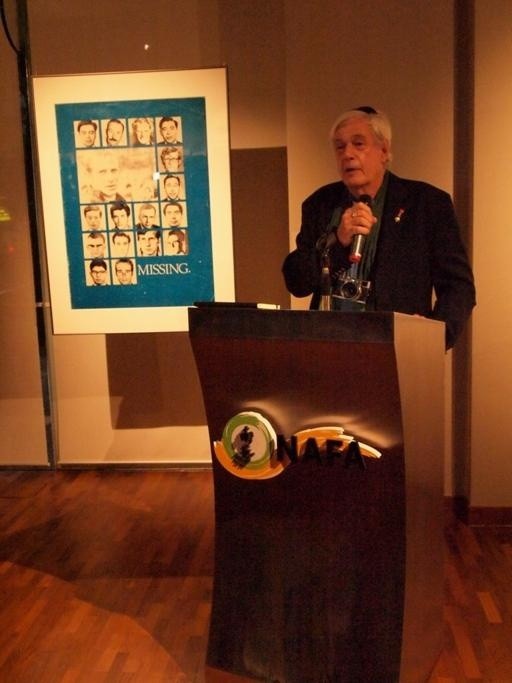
[331,276,372,305]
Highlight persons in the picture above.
[280,104,477,353]
[76,117,189,287]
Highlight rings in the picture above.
[350,210,356,217]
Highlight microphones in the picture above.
[348,193,372,263]
